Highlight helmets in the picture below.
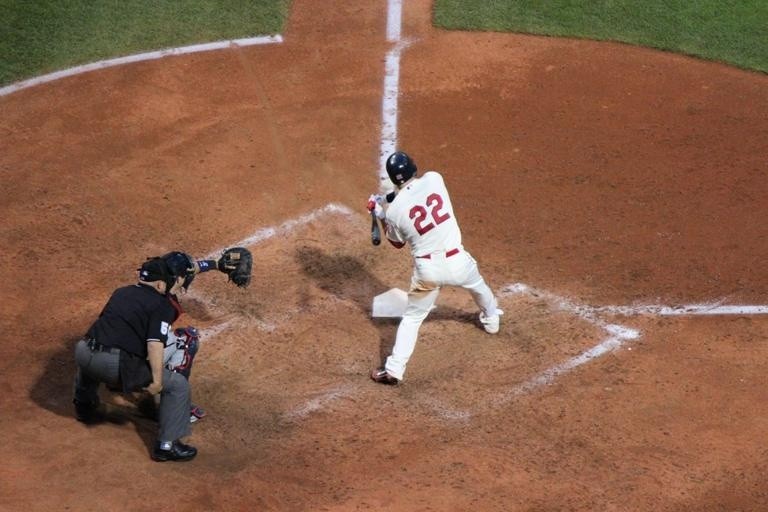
[162,252,196,290]
[386,151,417,186]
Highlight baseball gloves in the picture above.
[219,247,252,289]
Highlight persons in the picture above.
[366,152,500,385]
[73,247,252,462]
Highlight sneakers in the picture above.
[72,396,119,422]
[152,437,198,463]
[480,310,499,334]
[372,367,401,386]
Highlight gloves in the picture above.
[367,194,387,220]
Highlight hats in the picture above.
[140,256,175,282]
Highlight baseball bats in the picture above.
[370,207,381,246]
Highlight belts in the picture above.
[423,248,459,258]
[84,337,112,352]
